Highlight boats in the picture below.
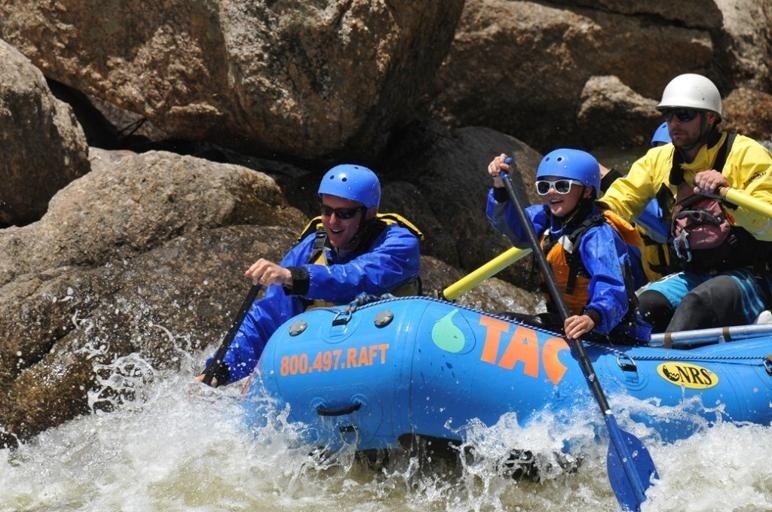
[241,296,771,461]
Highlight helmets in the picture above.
[317,163,382,208]
[656,73,723,114]
[536,148,600,199]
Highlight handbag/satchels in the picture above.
[669,183,734,251]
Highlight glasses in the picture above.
[660,109,699,121]
[319,204,363,219]
[535,179,583,196]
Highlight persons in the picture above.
[597,121,673,281]
[187,164,423,394]
[485,147,634,342]
[598,74,772,331]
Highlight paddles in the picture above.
[495,157,660,512]
